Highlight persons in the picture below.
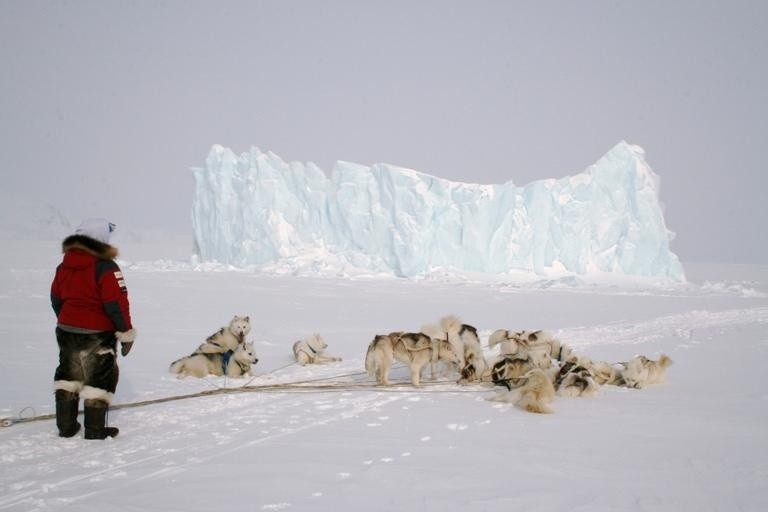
[51,217,137,440]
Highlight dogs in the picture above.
[428,316,488,386]
[169,340,259,380]
[190,314,252,355]
[483,369,555,415]
[293,331,344,367]
[487,329,672,389]
[365,330,461,389]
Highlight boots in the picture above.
[84,398,119,439]
[55,389,81,437]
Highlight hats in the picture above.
[77,218,115,242]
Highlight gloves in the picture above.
[120,340,133,356]
